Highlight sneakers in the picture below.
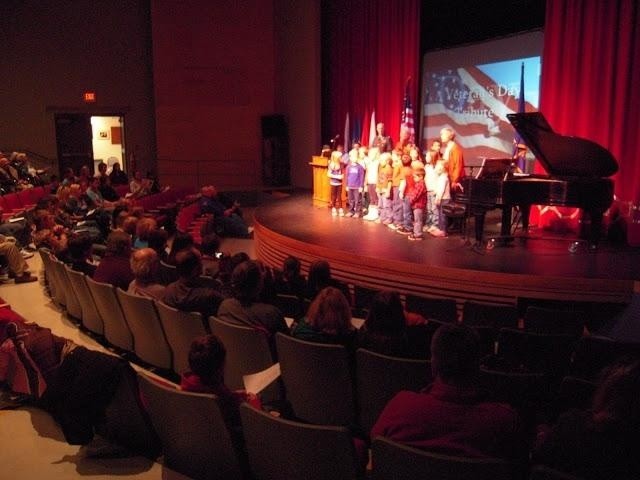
[386,221,447,242]
[331,205,393,224]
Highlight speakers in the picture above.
[261,114,289,187]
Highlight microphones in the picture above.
[330,134,340,143]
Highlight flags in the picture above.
[419,65,538,177]
[342,109,375,150]
[400,75,415,147]
[508,63,528,177]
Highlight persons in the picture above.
[1,152,256,479]
[328,123,465,240]
[220,255,640,479]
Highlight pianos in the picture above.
[463,112,618,256]
